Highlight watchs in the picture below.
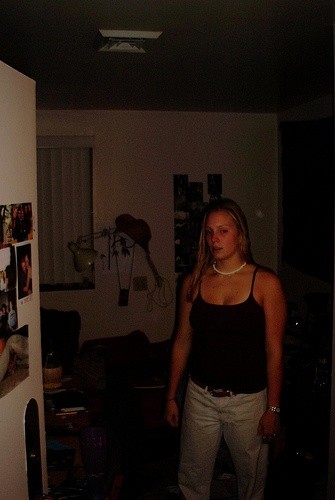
[268,405,281,415]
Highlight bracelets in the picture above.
[166,398,175,402]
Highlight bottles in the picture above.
[42,353,62,391]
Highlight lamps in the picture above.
[67,228,115,274]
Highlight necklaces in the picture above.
[211,259,247,276]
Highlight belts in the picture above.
[190,377,235,397]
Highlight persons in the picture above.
[163,199,281,500]
[0,204,32,336]
